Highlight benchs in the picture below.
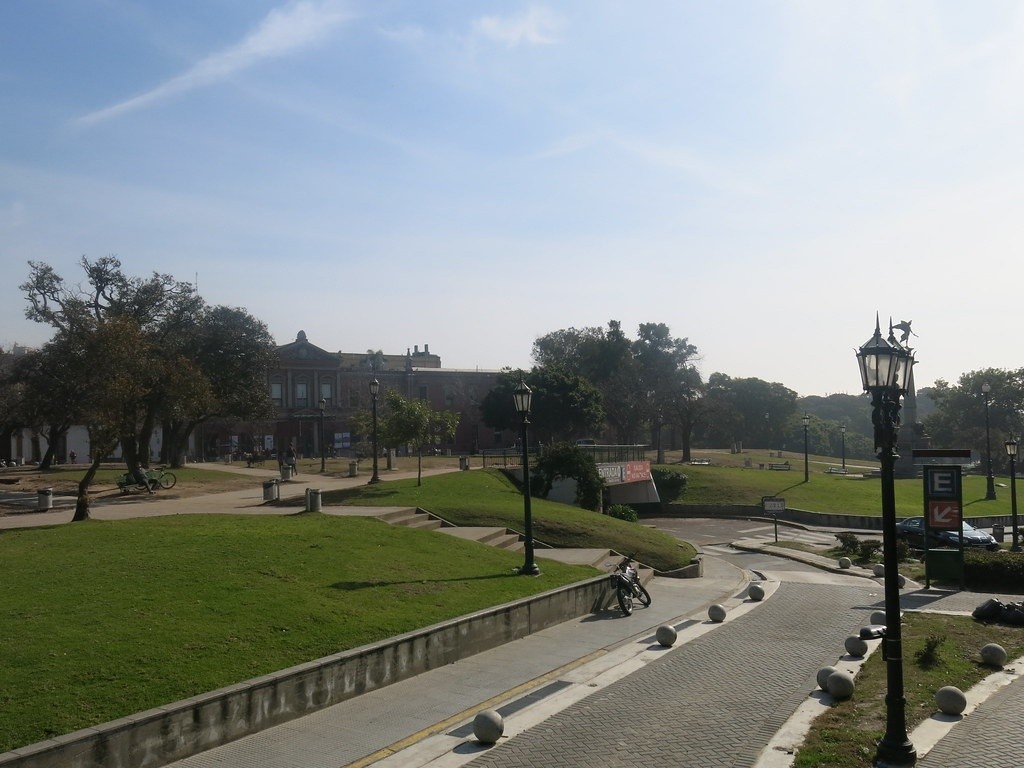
[114,472,159,496]
[769,463,791,471]
[825,466,849,476]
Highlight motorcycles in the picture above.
[604,550,651,616]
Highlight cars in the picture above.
[895,516,1000,557]
[575,439,596,446]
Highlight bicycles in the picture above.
[135,466,177,490]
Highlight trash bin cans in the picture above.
[992,524,1005,542]
[281,465,292,480]
[305,489,323,513]
[263,481,276,500]
[37,488,53,509]
[459,455,469,470]
[348,463,358,475]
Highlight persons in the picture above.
[210,443,298,477]
[69,450,77,464]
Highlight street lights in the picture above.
[1004,432,1022,552]
[764,412,770,447]
[319,397,326,471]
[839,423,847,470]
[368,378,379,480]
[802,410,810,481]
[855,311,916,768]
[981,379,997,501]
[511,373,540,578]
[656,414,664,463]
